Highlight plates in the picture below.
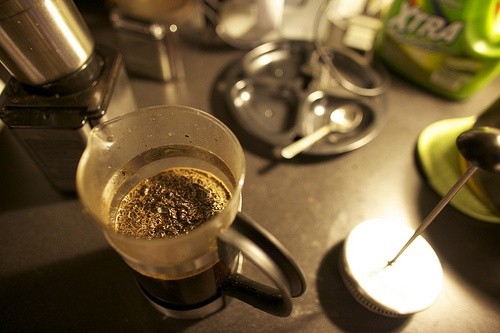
[213,35,388,163]
[419,114,500,224]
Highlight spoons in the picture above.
[281,103,364,160]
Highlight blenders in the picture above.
[0,0,139,201]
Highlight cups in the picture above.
[77,105,307,321]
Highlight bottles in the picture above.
[376,0,500,102]
